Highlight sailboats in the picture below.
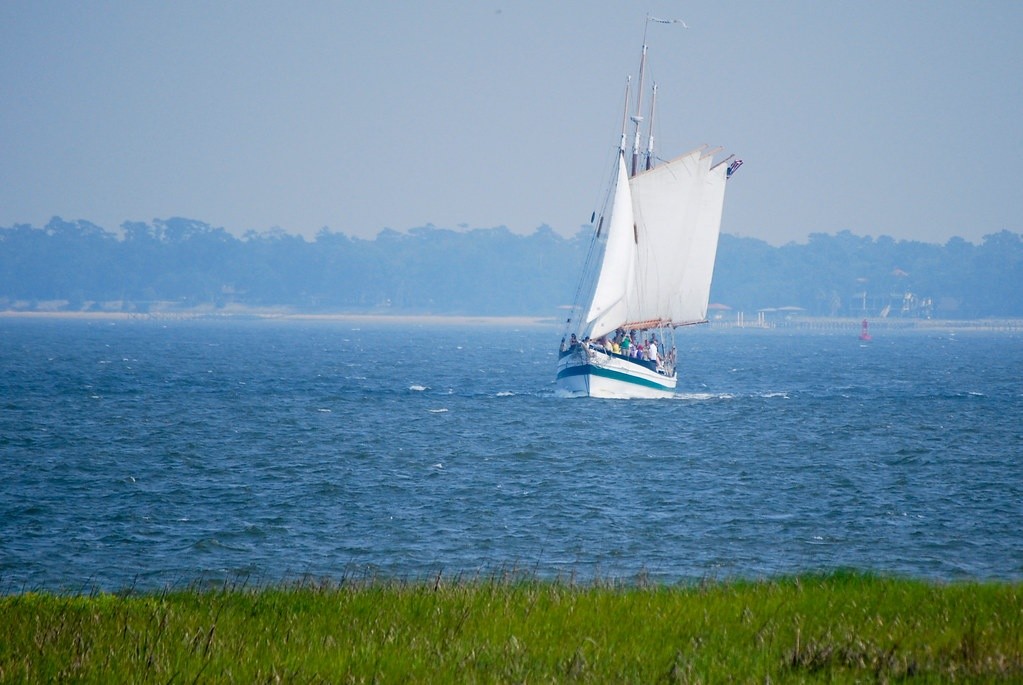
[557,14,743,398]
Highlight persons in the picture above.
[582,327,672,369]
[560,339,567,352]
[570,334,579,349]
